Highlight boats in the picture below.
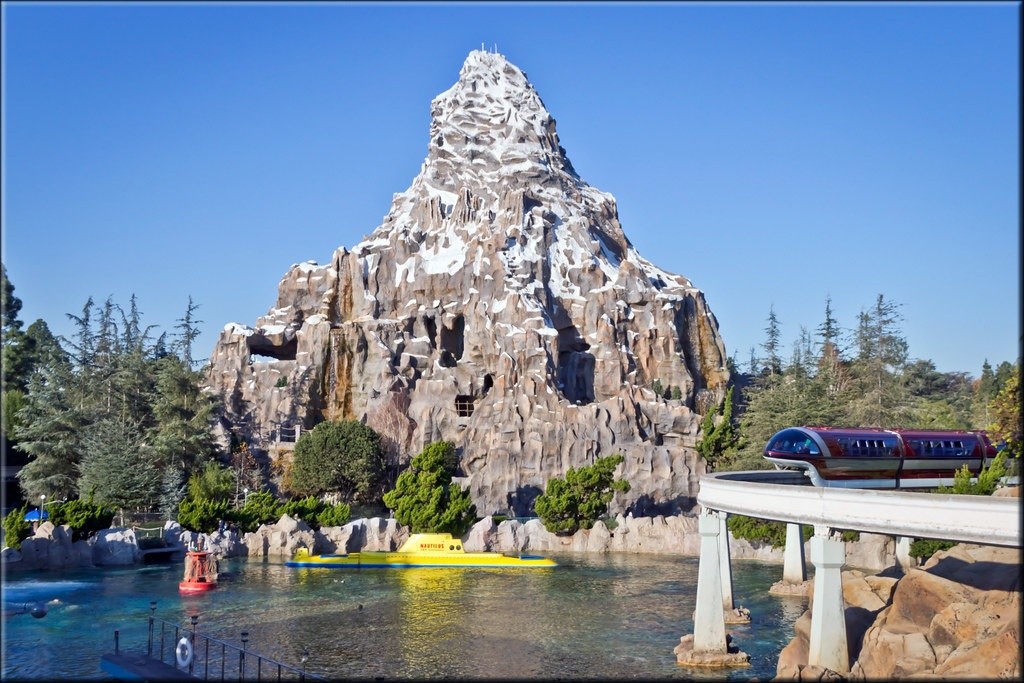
[179,541,218,596]
[283,532,559,568]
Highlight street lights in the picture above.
[39,492,68,528]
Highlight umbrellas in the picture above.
[24,508,49,522]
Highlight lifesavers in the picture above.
[176,637,192,666]
